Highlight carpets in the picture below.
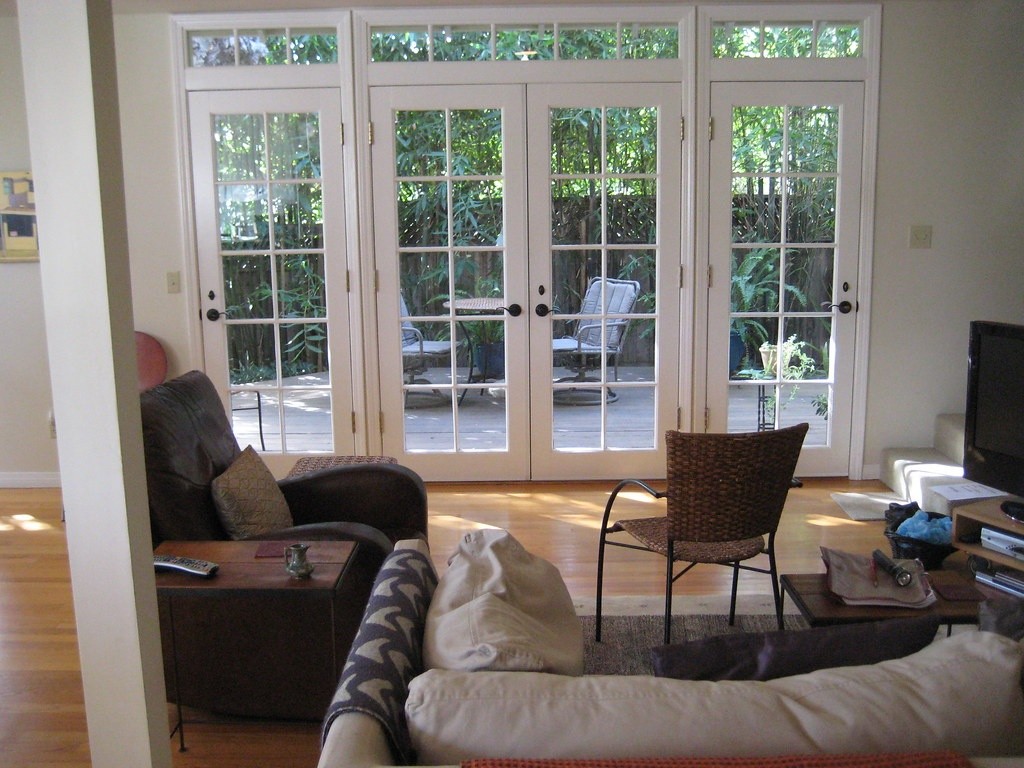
[578,614,812,676]
[831,491,910,522]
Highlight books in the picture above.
[975,567,1024,598]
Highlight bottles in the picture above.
[284,543,315,580]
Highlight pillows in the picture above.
[209,443,294,540]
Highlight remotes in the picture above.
[154,554,219,579]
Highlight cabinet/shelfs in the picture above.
[952,494,1024,572]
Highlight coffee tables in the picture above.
[776,570,989,632]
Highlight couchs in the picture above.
[315,529,1024,768]
[139,368,430,721]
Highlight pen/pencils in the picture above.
[869,559,879,587]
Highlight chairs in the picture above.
[553,276,641,406]
[400,295,463,410]
[592,421,810,649]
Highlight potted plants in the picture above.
[737,333,816,424]
[424,272,504,378]
[620,237,807,375]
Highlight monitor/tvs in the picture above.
[962,320,1024,524]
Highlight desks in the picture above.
[284,454,397,480]
[441,297,504,406]
[728,379,832,431]
[152,539,361,728]
